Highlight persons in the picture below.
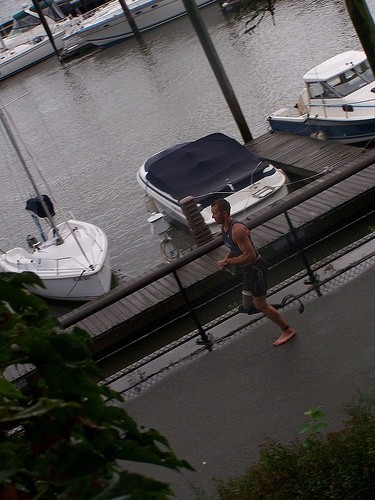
[210,198,297,346]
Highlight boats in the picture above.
[136,132,289,235]
[266,50,375,144]
[0,102,111,301]
[0,0,248,80]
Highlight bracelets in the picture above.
[224,261,228,268]
